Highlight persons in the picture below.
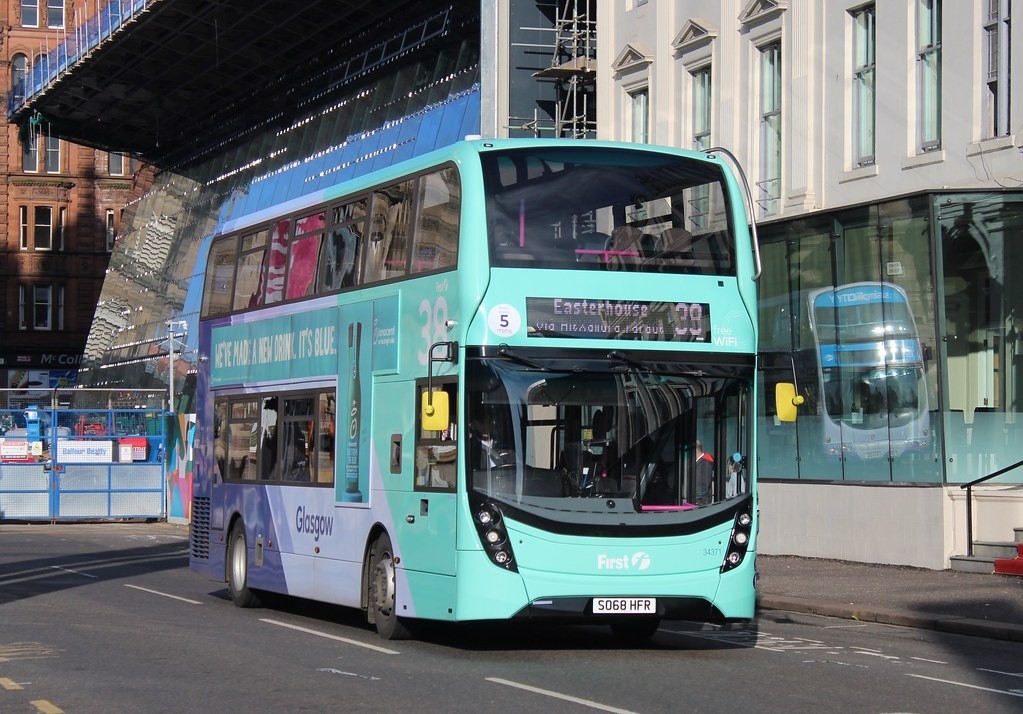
[469,420,506,470]
[726,455,745,498]
[830,379,899,414]
[694,440,712,506]
[440,429,452,441]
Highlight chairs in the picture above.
[493,215,690,271]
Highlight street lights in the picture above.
[165,320,188,412]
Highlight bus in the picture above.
[190,136,804,640]
[760,279,936,478]
